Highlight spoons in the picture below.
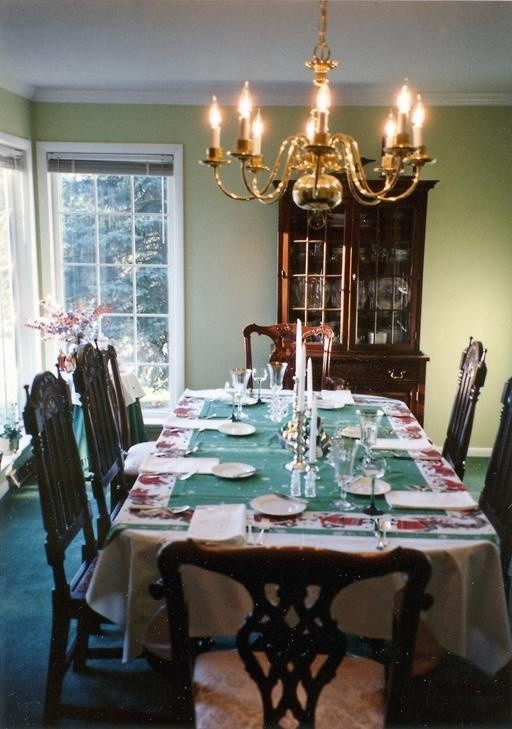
[129,503,190,514]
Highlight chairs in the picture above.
[21,341,211,728]
[419,336,512,729]
[139,540,438,728]
[243,321,335,392]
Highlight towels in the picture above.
[187,503,249,546]
[161,416,232,431]
[182,388,232,402]
[320,389,355,406]
[384,489,481,511]
[372,436,434,452]
[139,455,219,475]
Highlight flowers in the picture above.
[24,297,114,346]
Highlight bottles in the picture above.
[291,272,411,312]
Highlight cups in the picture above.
[265,361,289,399]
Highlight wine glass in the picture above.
[229,366,252,420]
[361,453,388,516]
[328,436,359,513]
[354,407,384,449]
[250,367,268,406]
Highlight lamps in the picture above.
[197,0,438,213]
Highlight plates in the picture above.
[219,395,258,407]
[342,478,391,497]
[249,492,308,518]
[209,461,256,480]
[218,420,257,437]
[317,399,346,410]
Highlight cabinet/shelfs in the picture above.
[268,344,430,431]
[270,177,440,356]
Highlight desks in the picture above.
[62,368,133,479]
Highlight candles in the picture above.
[294,318,318,464]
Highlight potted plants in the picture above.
[0,402,24,452]
